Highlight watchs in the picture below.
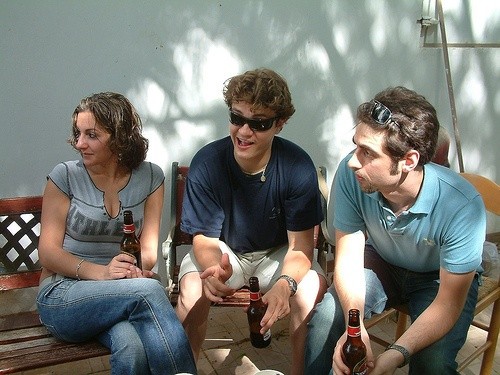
[276,275,297,297]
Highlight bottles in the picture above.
[342,309,367,375]
[120,210,143,279]
[247,277,271,349]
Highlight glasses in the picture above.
[368,98,416,148]
[228,108,282,132]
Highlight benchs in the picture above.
[0,194,113,375]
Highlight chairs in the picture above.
[163,161,328,307]
[359,173,500,375]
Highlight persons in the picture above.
[176,69,330,375]
[36,91,197,375]
[304,85,487,375]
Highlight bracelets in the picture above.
[76,259,86,280]
[385,344,410,368]
